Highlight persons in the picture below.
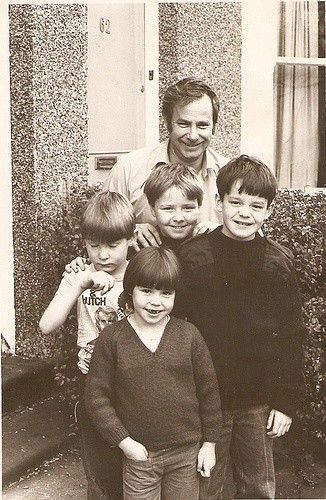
[171,155,308,500]
[127,162,204,262]
[102,77,235,260]
[38,192,135,499]
[83,246,223,500]
